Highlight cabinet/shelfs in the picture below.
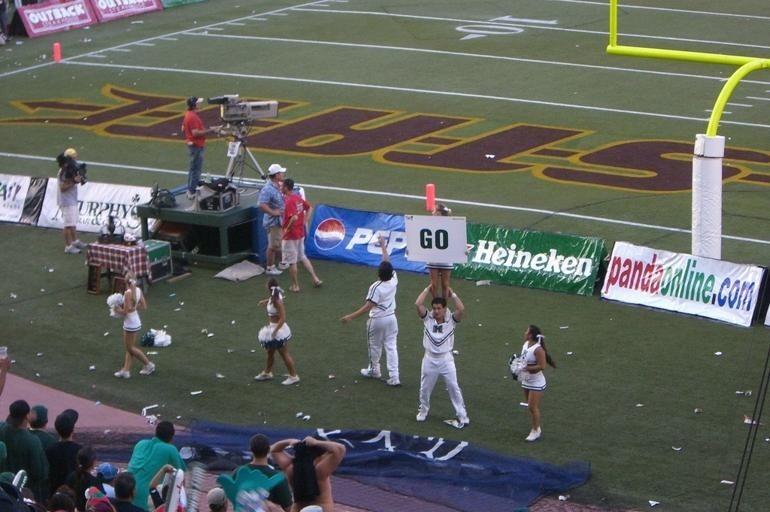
[136,183,302,270]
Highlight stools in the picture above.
[111,275,127,294]
[143,238,173,283]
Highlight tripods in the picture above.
[219,136,267,188]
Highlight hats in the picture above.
[54,408,78,434]
[187,96,204,106]
[31,405,48,428]
[6,400,31,424]
[268,163,287,175]
[64,148,76,158]
[207,488,226,508]
[96,462,116,479]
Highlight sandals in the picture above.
[289,280,322,291]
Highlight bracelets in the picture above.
[451,291,457,299]
[423,287,430,294]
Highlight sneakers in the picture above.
[139,360,155,376]
[114,370,130,378]
[388,378,400,384]
[525,426,541,441]
[281,375,299,385]
[429,310,434,318]
[266,262,290,275]
[186,190,197,200]
[444,308,451,322]
[461,418,471,424]
[64,240,88,253]
[253,371,273,380]
[416,411,428,420]
[360,369,382,379]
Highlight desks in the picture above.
[86,241,152,296]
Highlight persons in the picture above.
[257,162,292,275]
[253,278,300,386]
[339,232,412,388]
[280,179,323,293]
[513,321,555,444]
[232,434,293,510]
[56,150,84,256]
[26,405,58,452]
[414,279,473,427]
[127,421,186,510]
[270,436,345,510]
[208,487,229,510]
[114,276,157,381]
[3,400,50,504]
[425,206,453,301]
[45,409,84,498]
[3,446,187,510]
[261,498,323,510]
[180,96,221,200]
